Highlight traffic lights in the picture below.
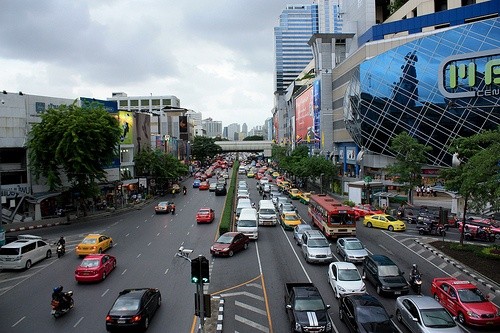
[190,257,211,285]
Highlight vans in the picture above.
[259,200,277,227]
[237,209,259,240]
[0,239,52,271]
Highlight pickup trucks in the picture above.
[285,281,333,333]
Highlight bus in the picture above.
[308,193,356,236]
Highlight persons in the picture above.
[55,235,65,254]
[379,185,456,226]
[52,286,73,312]
[80,181,187,215]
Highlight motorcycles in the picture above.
[408,273,424,295]
[54,242,65,258]
[401,202,500,242]
[51,292,74,318]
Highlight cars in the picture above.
[210,232,250,257]
[396,295,460,333]
[195,208,215,222]
[105,288,161,333]
[362,254,409,296]
[154,200,173,214]
[431,277,499,327]
[457,216,500,234]
[300,230,333,265]
[337,237,367,264]
[293,224,311,245]
[363,215,406,232]
[75,233,113,256]
[74,253,117,283]
[339,293,396,333]
[326,262,365,298]
[192,150,236,195]
[237,151,313,230]
[351,204,381,217]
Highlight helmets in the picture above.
[412,263,417,270]
[54,286,63,293]
